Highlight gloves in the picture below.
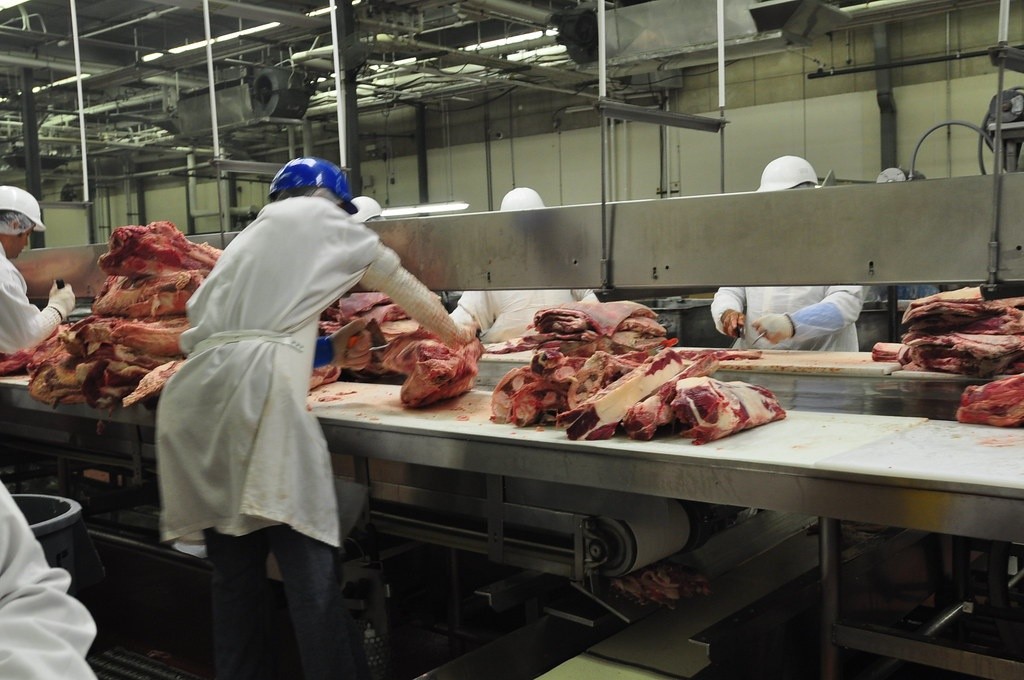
[327,318,371,371]
[47,279,75,320]
[752,313,794,344]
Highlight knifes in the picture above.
[55,278,77,327]
[738,326,748,349]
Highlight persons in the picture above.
[0,481,99,680]
[0,186,76,353]
[449,186,599,344]
[155,157,474,680]
[711,155,870,352]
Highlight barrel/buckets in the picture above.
[10,491,112,658]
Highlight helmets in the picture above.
[348,196,382,222]
[0,186,47,232]
[268,156,358,214]
[756,155,818,192]
[500,187,545,211]
